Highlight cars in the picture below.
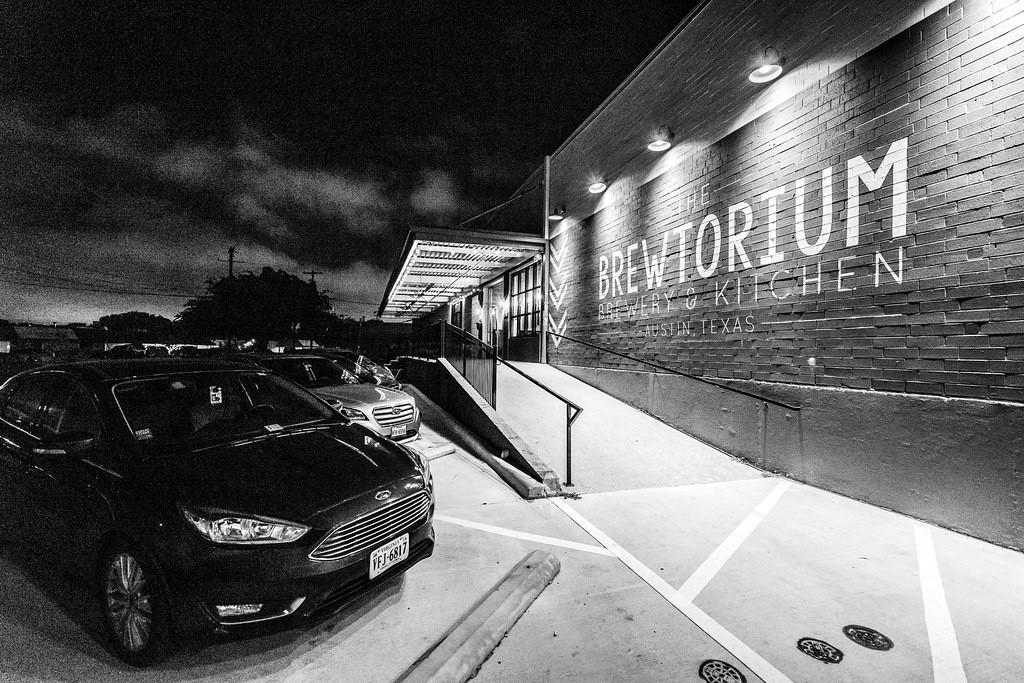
[0,357,436,667]
[105,342,422,442]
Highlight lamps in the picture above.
[548,203,565,220]
[648,125,674,151]
[748,46,786,83]
[588,173,607,193]
